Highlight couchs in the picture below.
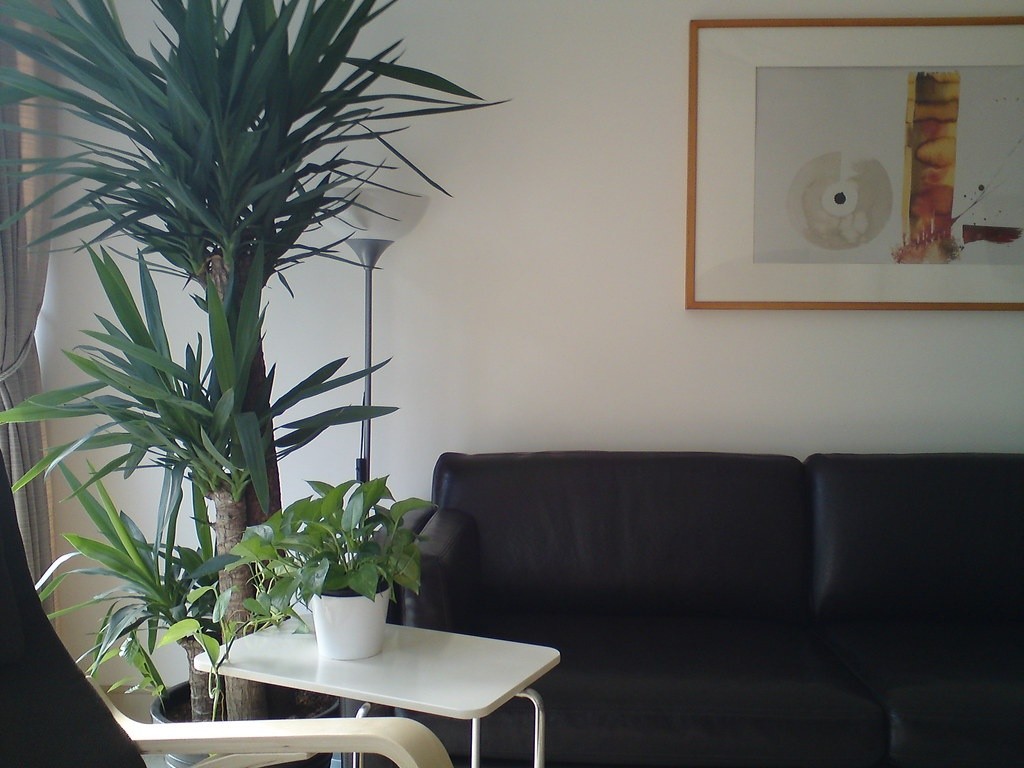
[340,451,1024,767]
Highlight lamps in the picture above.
[323,187,429,481]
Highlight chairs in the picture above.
[0,449,454,768]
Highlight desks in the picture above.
[194,613,560,768]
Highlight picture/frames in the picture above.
[685,16,1024,310]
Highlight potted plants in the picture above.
[155,474,439,722]
[0,0,513,768]
